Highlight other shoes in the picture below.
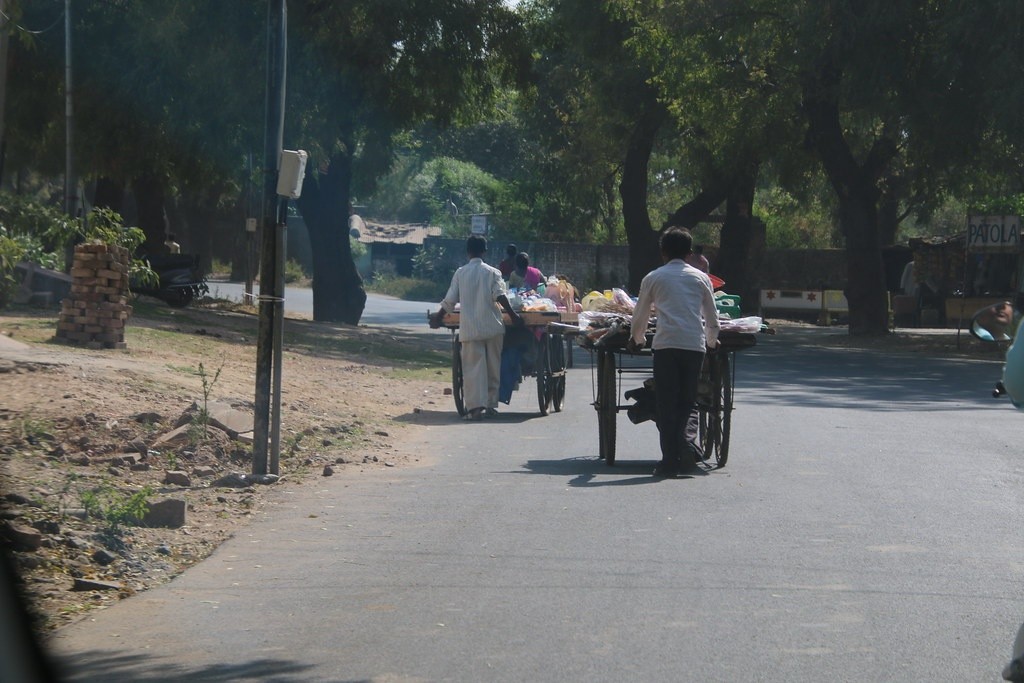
[681,446,697,472]
[653,465,676,477]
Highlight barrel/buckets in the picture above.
[714,295,741,319]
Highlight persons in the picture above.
[1001,318,1024,410]
[628,225,721,475]
[428,235,521,421]
[509,252,547,291]
[689,244,709,273]
[975,304,1013,340]
[500,244,517,279]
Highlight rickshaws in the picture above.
[426,295,584,417]
[548,316,777,466]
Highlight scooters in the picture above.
[136,251,199,307]
